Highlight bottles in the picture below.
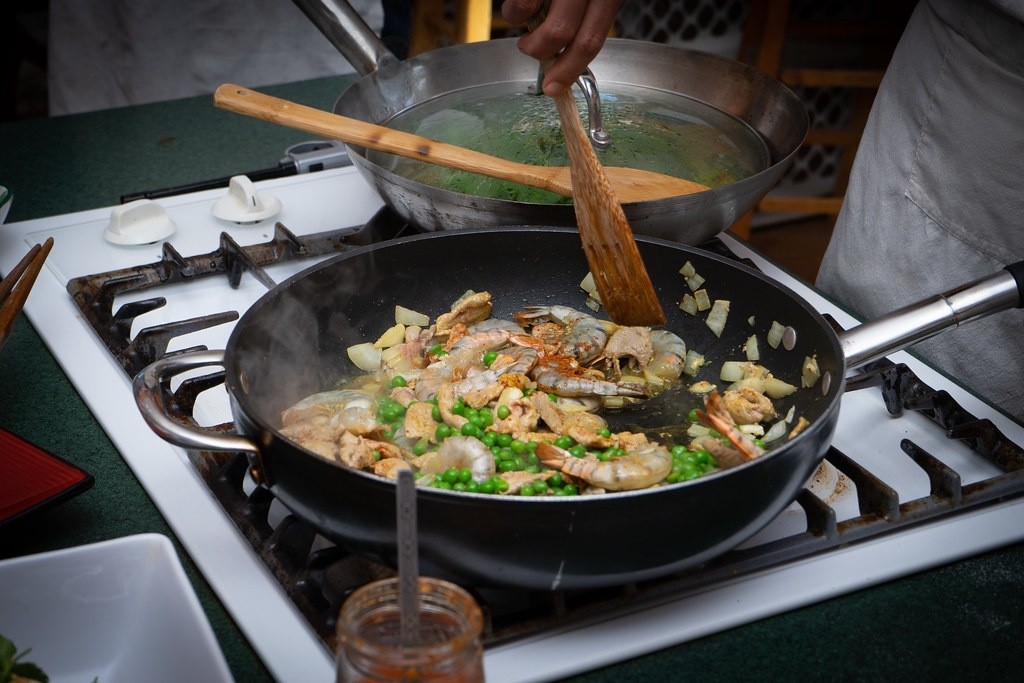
[333,572,484,683]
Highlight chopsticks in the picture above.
[0,237,55,356]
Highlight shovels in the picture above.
[528,8,666,330]
[212,82,708,205]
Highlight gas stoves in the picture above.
[1,164,1023,683]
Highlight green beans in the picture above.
[374,346,765,495]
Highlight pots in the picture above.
[129,224,1021,590]
[293,1,813,251]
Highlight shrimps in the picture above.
[275,305,766,491]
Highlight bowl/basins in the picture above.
[0,531,237,683]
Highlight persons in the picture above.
[503,0,1024,431]
